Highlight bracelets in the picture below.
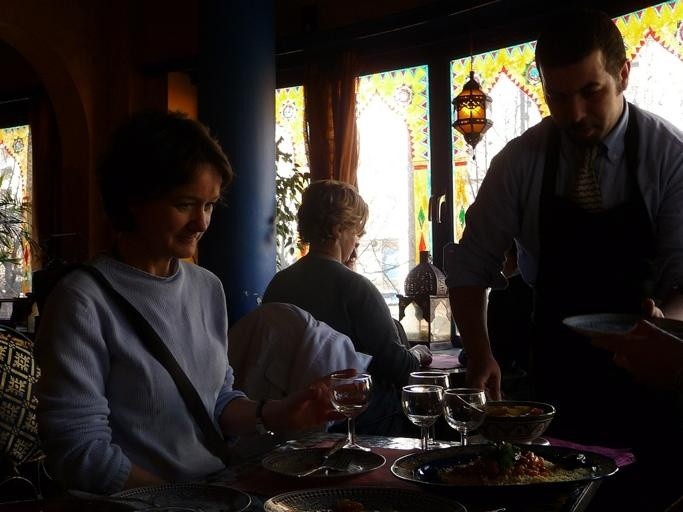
[253,396,273,438]
[410,347,423,367]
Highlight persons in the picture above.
[34,107,368,496]
[439,6,681,445]
[456,233,537,397]
[588,316,682,382]
[260,178,434,440]
[344,226,368,272]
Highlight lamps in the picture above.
[452,44,495,150]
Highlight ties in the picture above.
[564,141,602,214]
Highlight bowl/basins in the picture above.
[473,401,555,444]
[264,486,466,511]
[391,443,618,510]
[561,313,682,362]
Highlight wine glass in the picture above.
[329,374,372,453]
[444,387,488,454]
[408,372,450,455]
[403,385,441,456]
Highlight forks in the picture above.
[295,456,351,477]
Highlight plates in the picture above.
[108,484,253,511]
[261,446,388,482]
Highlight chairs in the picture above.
[0,320,57,511]
